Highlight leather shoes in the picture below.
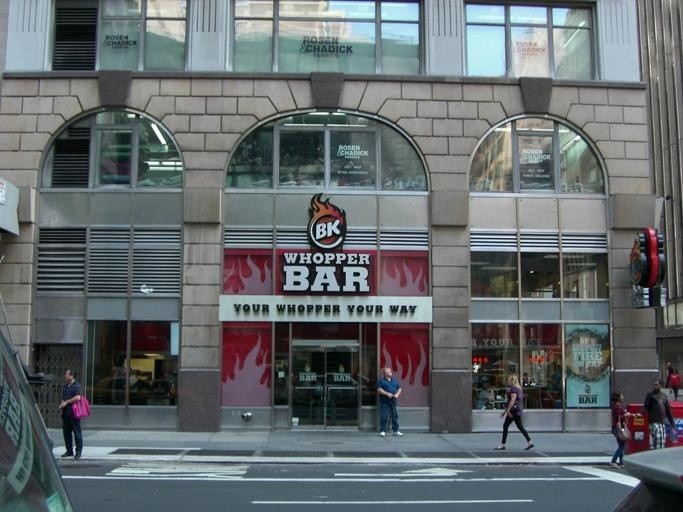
[75,455,81,458]
[61,450,73,457]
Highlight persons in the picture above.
[522,371,536,387]
[374,366,404,437]
[493,374,535,449]
[635,379,676,451]
[663,360,674,388]
[669,366,680,402]
[57,368,83,460]
[474,379,495,410]
[548,364,562,393]
[608,388,637,471]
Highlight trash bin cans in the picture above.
[665,399,683,447]
[625,403,650,454]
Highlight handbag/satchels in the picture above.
[71,396,91,420]
[617,423,632,440]
[510,404,519,413]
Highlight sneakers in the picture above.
[392,431,403,436]
[380,432,385,437]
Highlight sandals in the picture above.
[524,445,534,450]
[495,447,506,449]
